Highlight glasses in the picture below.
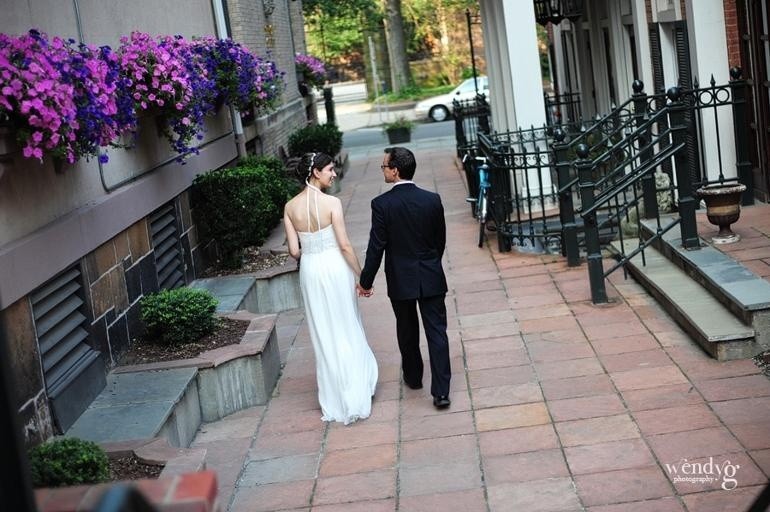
[380,165,392,169]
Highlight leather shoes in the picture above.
[432,396,450,412]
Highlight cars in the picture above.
[415,75,490,122]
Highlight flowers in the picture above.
[0,27,329,168]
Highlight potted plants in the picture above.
[379,116,415,144]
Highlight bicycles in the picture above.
[461,153,496,248]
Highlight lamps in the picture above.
[534,0,586,28]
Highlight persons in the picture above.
[282,151,379,426]
[355,146,452,410]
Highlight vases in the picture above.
[695,181,747,245]
